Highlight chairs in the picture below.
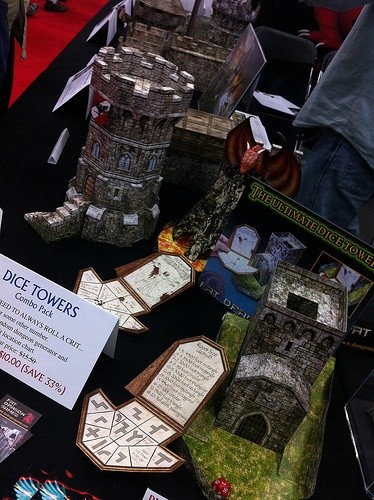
[245,26,315,146]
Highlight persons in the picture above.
[0,0,28,115]
[291,1,374,240]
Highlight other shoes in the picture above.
[44,0,68,12]
